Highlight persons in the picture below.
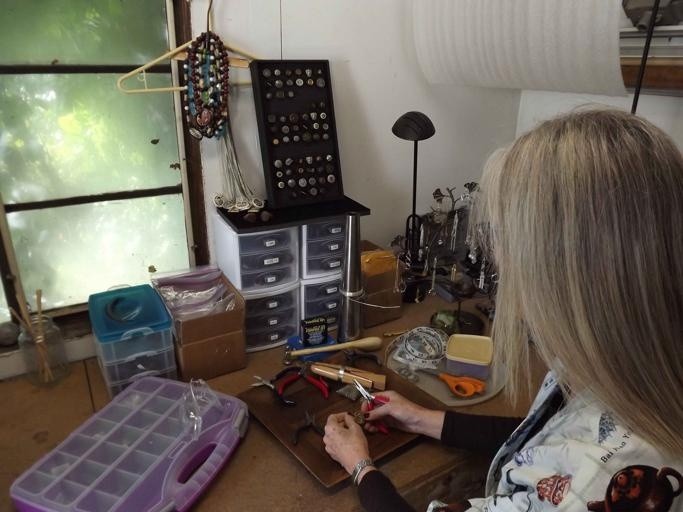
[323,103,682,512]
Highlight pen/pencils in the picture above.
[9,280,66,384]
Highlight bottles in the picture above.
[17,315,68,386]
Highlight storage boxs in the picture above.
[210,211,403,353]
[87,260,247,403]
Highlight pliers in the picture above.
[248,361,329,446]
[342,349,381,368]
[353,378,396,436]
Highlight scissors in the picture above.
[423,368,486,398]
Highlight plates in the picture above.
[235,350,452,489]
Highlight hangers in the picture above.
[114,1,261,94]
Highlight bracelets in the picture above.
[350,459,376,488]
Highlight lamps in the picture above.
[390,111,436,304]
